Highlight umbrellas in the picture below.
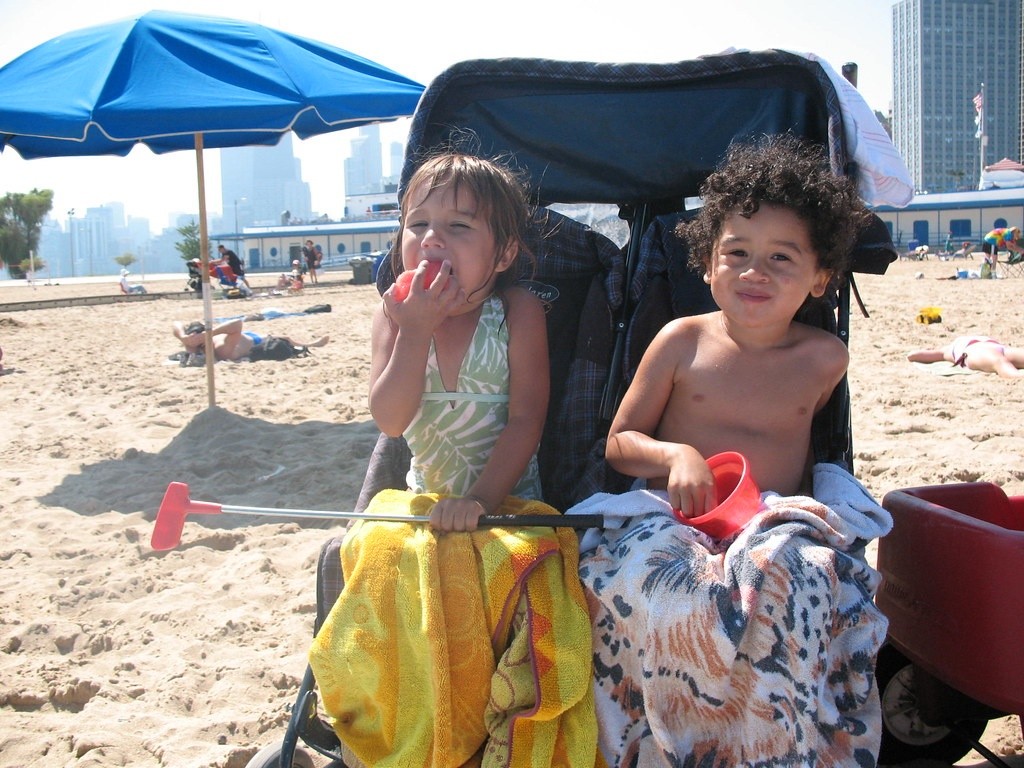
[1,7,428,406]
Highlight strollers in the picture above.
[258,48,899,768]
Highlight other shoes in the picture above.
[312,280,317,284]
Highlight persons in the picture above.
[172,318,331,361]
[906,244,928,256]
[945,230,953,255]
[907,334,1024,380]
[982,226,1024,279]
[366,154,552,535]
[279,260,304,291]
[304,239,323,285]
[120,269,147,295]
[604,129,849,539]
[192,244,253,291]
[956,242,968,255]
[213,309,307,323]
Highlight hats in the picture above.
[121,269,129,276]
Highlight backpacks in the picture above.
[250,335,310,362]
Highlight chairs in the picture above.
[900,242,976,261]
[997,238,1024,278]
[120,282,132,295]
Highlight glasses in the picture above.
[293,263,297,265]
